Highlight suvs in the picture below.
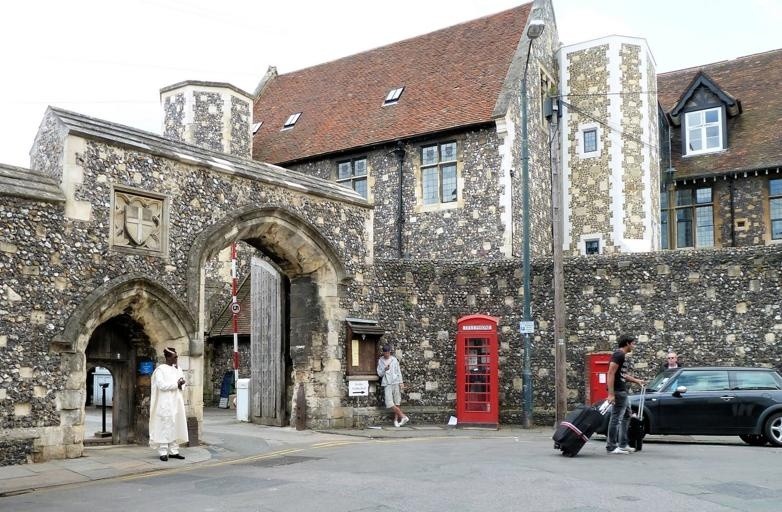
[588,366,782,446]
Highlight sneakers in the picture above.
[605,445,637,457]
[393,416,410,427]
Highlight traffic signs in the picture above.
[229,301,242,314]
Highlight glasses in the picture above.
[169,356,178,358]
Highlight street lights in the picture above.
[520,19,547,428]
[663,165,678,254]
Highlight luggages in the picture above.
[553,404,602,457]
[626,413,648,451]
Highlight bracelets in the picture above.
[608,386,614,392]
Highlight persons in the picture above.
[606,333,646,454]
[147,347,189,461]
[376,346,410,427]
[654,352,685,392]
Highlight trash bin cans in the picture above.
[236,378,251,423]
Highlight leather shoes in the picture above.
[160,454,168,461]
[169,453,186,460]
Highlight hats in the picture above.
[383,346,391,352]
